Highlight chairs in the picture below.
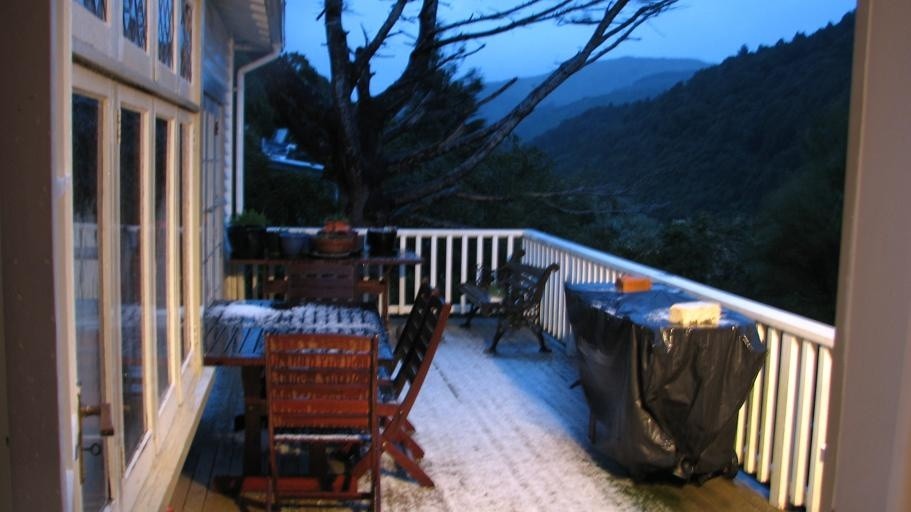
[285,261,358,306]
[264,331,380,511]
[313,277,452,487]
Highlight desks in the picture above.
[231,251,425,326]
[199,299,392,477]
[564,284,764,487]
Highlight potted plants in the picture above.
[225,208,267,259]
[310,211,362,258]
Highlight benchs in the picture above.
[457,247,559,353]
